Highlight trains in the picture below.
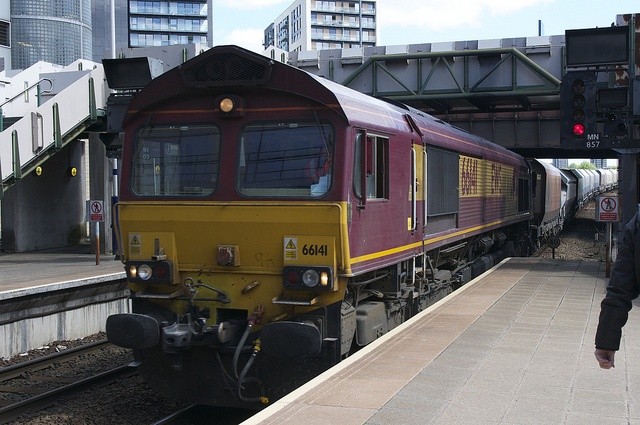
[105,46,617,411]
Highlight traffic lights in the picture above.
[559,72,595,148]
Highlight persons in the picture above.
[594,203,638,370]
